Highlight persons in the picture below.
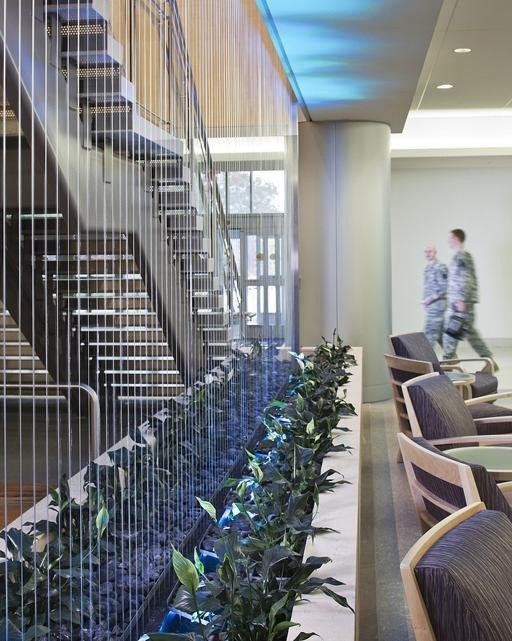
[421,229,499,374]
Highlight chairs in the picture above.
[383,331,511,641]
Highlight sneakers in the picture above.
[477,362,498,374]
